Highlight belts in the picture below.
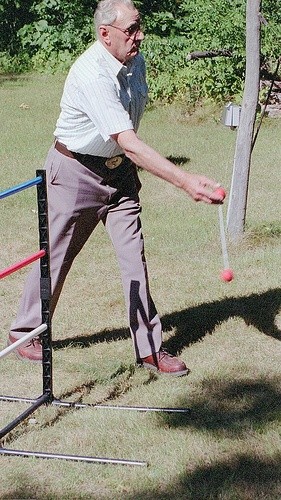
[55,141,126,169]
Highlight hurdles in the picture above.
[0,168,192,468]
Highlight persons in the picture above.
[8,0,223,377]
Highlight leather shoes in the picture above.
[8,333,44,361]
[136,346,189,378]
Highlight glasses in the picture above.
[105,23,144,37]
